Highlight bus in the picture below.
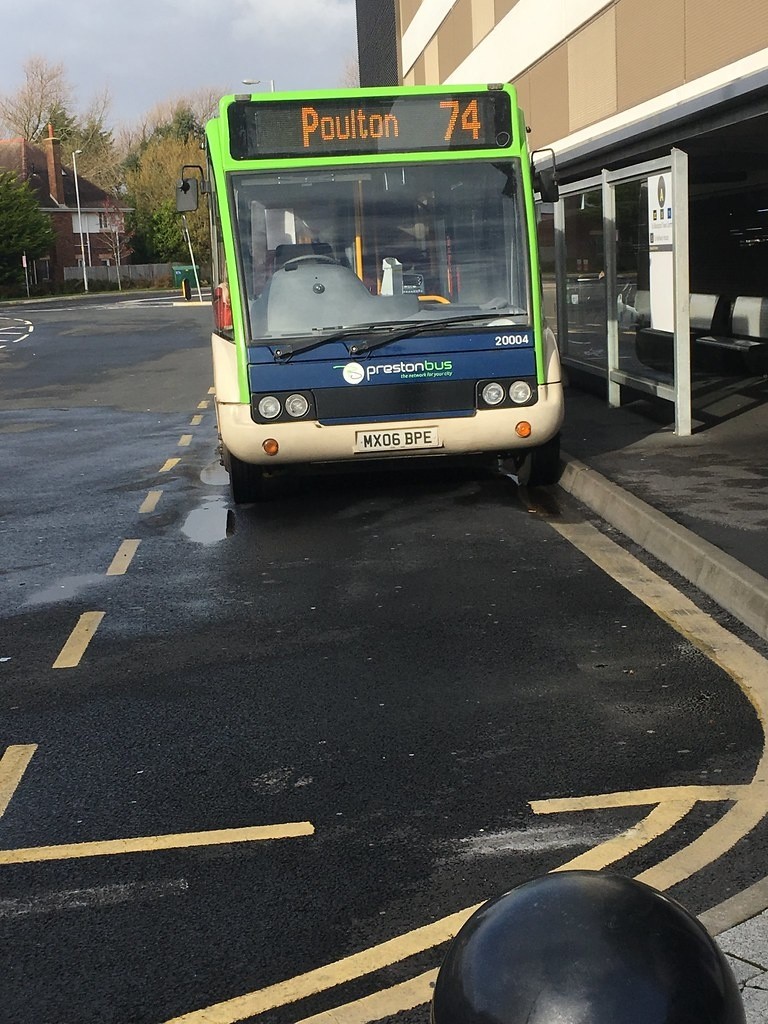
[172,82,559,508]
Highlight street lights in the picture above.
[241,78,276,93]
[70,149,89,296]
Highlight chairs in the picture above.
[273,242,335,273]
[619,289,768,353]
